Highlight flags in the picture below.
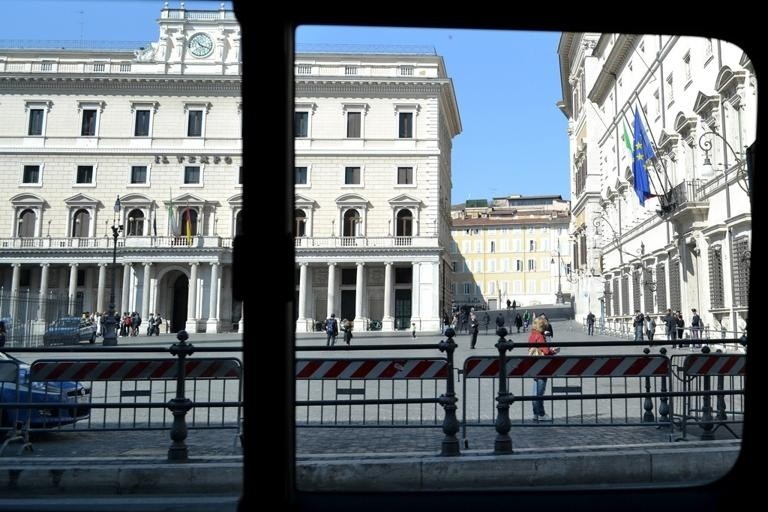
[633,104,656,207]
[622,126,634,155]
[167,199,182,240]
[186,204,193,246]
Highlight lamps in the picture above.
[592,215,621,253]
[699,132,749,196]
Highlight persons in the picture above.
[81,312,142,337]
[587,312,595,336]
[441,312,449,335]
[322,313,341,345]
[513,312,522,334]
[340,319,353,346]
[532,311,537,322]
[538,312,546,319]
[631,308,702,349]
[147,313,155,336]
[411,323,418,339]
[512,300,516,310]
[482,311,491,334]
[469,315,479,350]
[522,309,530,332]
[528,316,559,421]
[154,312,161,335]
[506,298,511,310]
[450,314,458,329]
[495,312,505,330]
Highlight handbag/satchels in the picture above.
[527,342,545,356]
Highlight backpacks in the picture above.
[326,319,336,333]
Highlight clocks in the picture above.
[187,32,214,59]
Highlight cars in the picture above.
[43,317,97,346]
[0,317,25,346]
[0,352,93,431]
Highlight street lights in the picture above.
[108,225,119,317]
[551,251,565,304]
